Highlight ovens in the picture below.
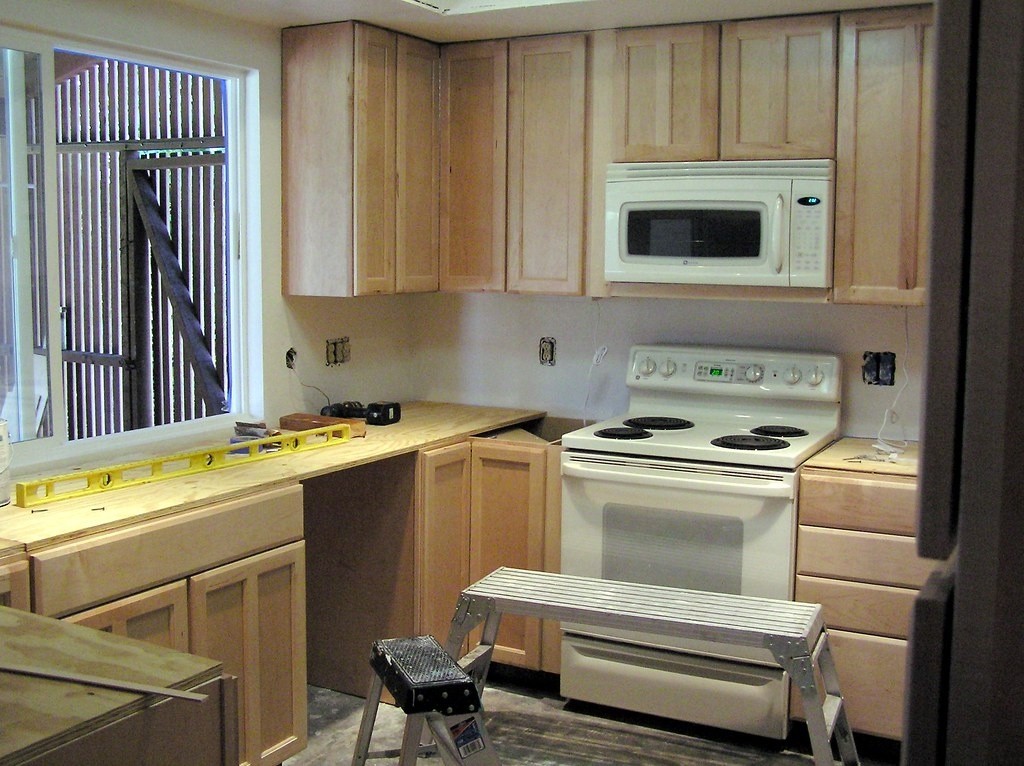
[560,452,799,740]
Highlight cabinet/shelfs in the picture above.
[302,443,472,709]
[790,436,941,742]
[833,6,935,309]
[472,438,549,672]
[608,12,837,163]
[29,480,311,766]
[281,19,442,296]
[440,31,589,295]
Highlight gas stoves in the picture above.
[562,345,843,469]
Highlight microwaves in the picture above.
[604,158,834,289]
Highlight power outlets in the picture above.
[538,337,556,367]
[326,336,351,365]
